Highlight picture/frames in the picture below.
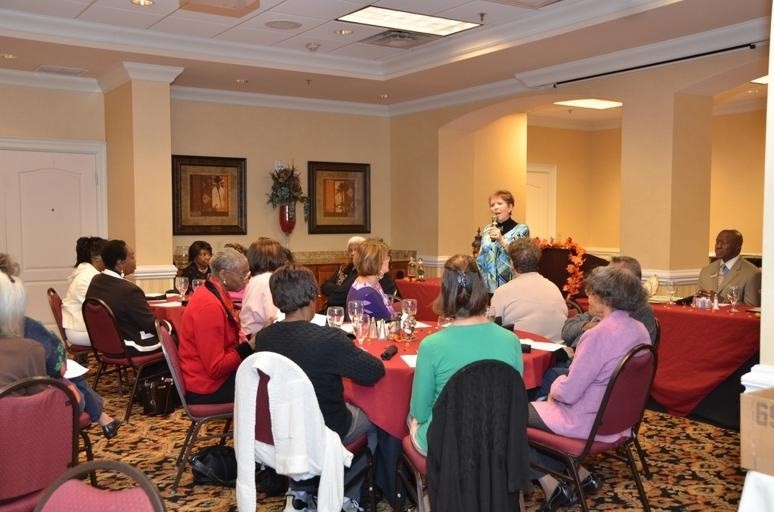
[307,161,372,235]
[172,154,248,236]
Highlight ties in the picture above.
[718,265,726,285]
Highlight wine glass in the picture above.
[326,301,370,353]
[174,277,190,302]
[666,279,678,306]
[727,284,740,313]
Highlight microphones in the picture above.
[490,216,499,243]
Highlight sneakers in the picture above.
[341,496,362,512]
[282,490,317,512]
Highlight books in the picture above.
[644,288,724,306]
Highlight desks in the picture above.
[131,287,249,368]
[576,296,760,434]
[395,276,445,322]
[328,319,568,462]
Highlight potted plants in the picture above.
[267,162,308,232]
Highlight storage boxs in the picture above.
[740,389,774,478]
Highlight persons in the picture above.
[692,229,761,310]
[85,239,184,409]
[0,252,121,440]
[474,191,530,307]
[60,235,108,348]
[176,232,661,512]
[0,270,48,398]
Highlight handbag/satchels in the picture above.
[139,370,178,417]
[255,462,288,497]
[191,444,237,485]
[359,471,408,512]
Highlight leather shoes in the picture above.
[576,473,600,493]
[102,418,122,440]
[534,480,578,512]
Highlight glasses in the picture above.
[225,271,251,280]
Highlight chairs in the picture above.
[531,341,662,510]
[45,288,101,372]
[80,295,168,412]
[153,315,236,495]
[78,413,100,487]
[232,351,374,512]
[627,317,661,480]
[401,362,530,511]
[33,458,167,512]
[0,376,98,511]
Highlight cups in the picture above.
[192,280,205,290]
[483,306,496,321]
[402,300,417,315]
[437,316,453,329]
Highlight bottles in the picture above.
[407,256,424,280]
[369,316,386,340]
[694,289,719,311]
